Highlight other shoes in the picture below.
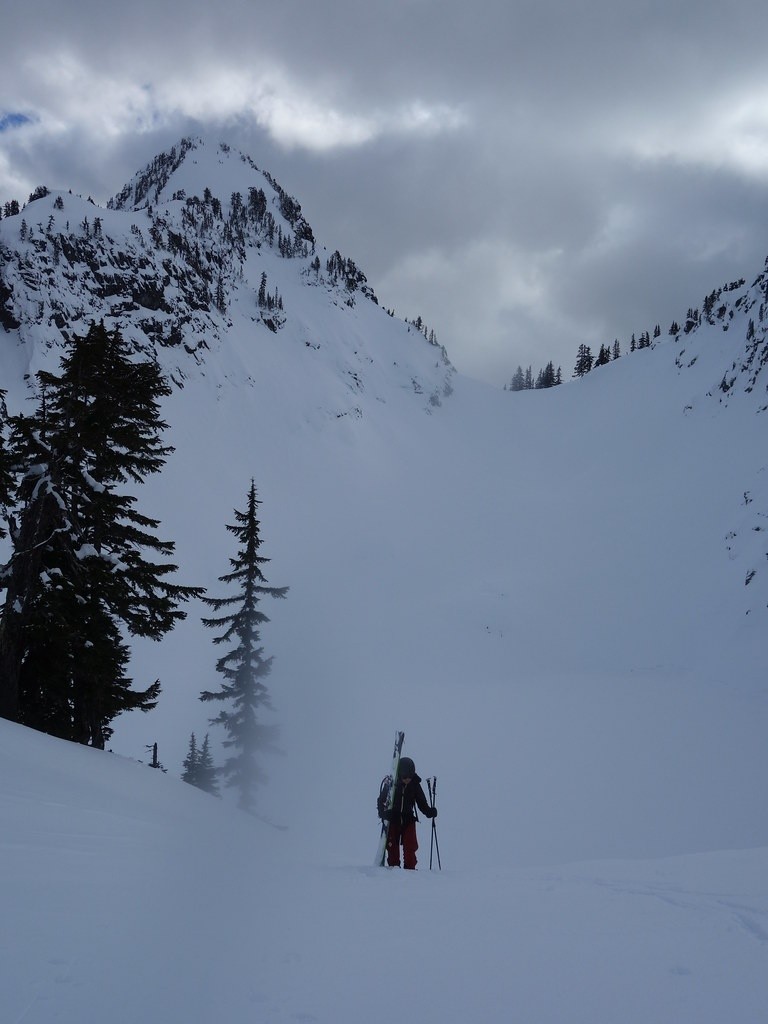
[389,864,401,868]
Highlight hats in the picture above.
[399,757,415,775]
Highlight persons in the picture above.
[377,757,438,870]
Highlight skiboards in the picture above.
[374,728,405,867]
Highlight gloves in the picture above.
[426,807,437,818]
[384,809,393,821]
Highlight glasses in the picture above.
[402,775,414,779]
